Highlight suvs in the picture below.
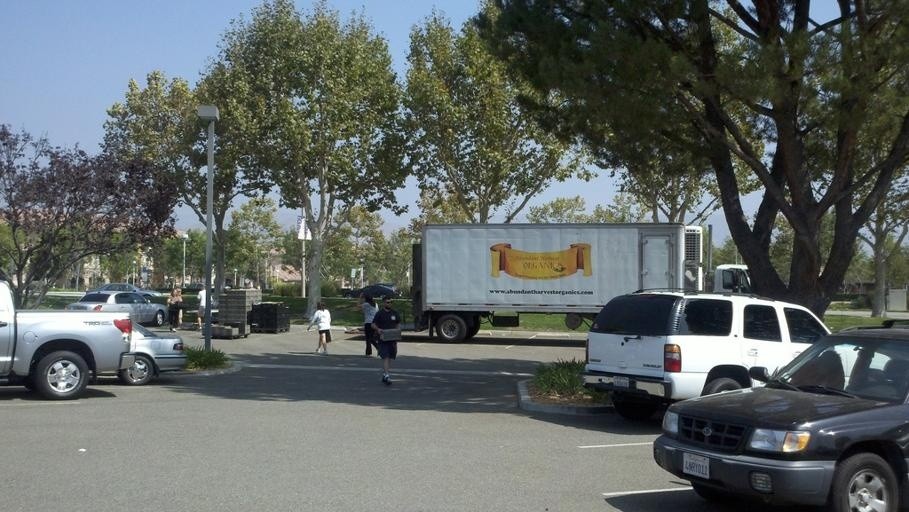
[579,288,892,421]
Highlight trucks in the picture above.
[412,224,754,344]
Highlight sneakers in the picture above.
[316,348,328,355]
[382,372,393,385]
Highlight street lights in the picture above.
[276,270,279,282]
[132,260,136,286]
[234,269,237,286]
[360,258,365,288]
[180,234,188,287]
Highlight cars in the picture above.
[652,316,909,512]
[343,284,399,299]
[181,283,215,294]
[64,283,188,386]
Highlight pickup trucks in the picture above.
[0,279,137,400]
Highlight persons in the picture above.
[360,290,380,359]
[370,296,402,385]
[167,281,214,333]
[307,301,332,356]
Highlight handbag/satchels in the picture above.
[326,330,332,343]
